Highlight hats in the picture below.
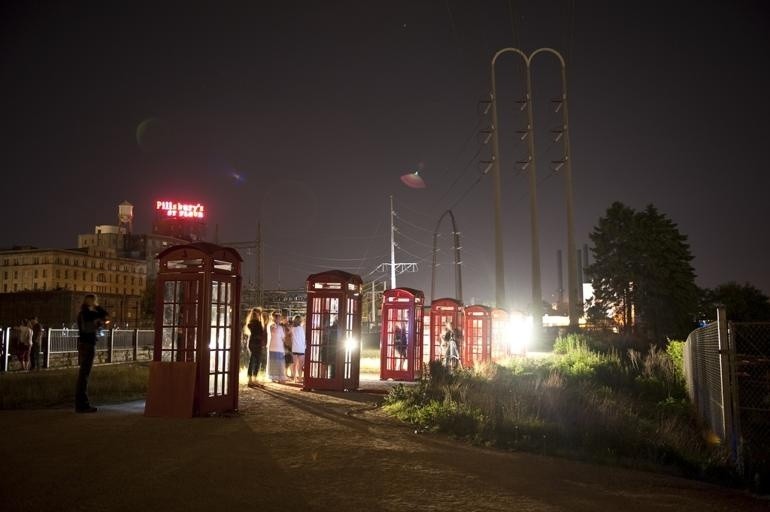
[271,310,282,316]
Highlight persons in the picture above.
[17,319,32,370]
[75,294,109,413]
[30,316,42,373]
[440,322,463,371]
[393,320,407,370]
[237,306,306,389]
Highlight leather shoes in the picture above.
[75,406,98,413]
[248,383,256,388]
[253,383,263,387]
